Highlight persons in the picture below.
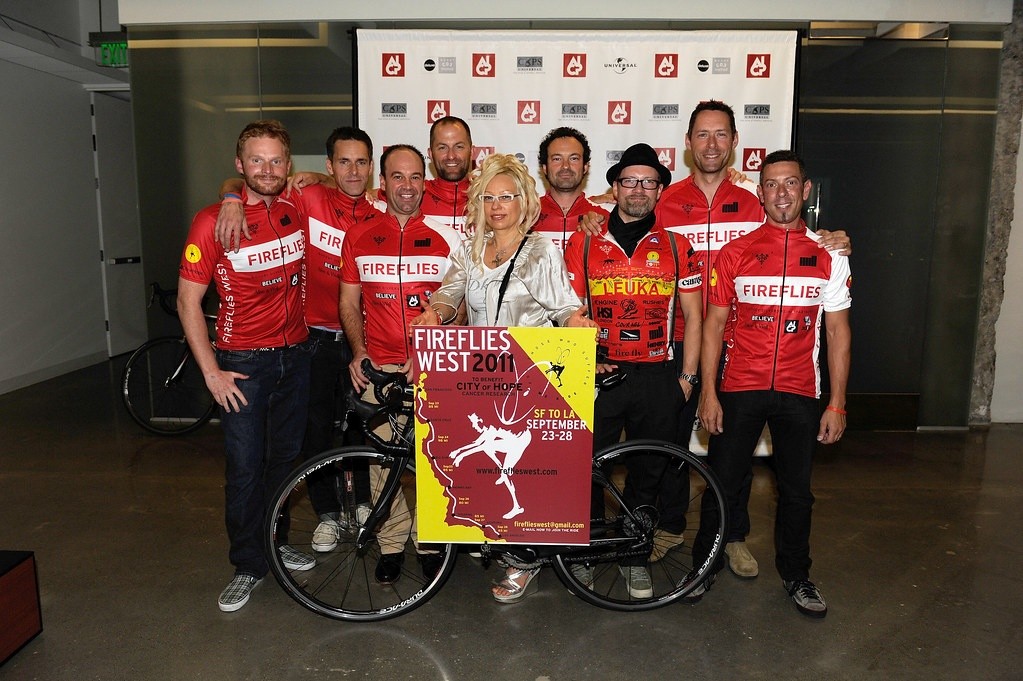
[178,100,853,610]
[340,145,465,587]
[563,142,703,598]
[677,150,852,616]
[408,154,600,602]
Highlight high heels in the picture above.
[493,564,541,604]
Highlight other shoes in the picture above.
[338,511,352,528]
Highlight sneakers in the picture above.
[276,545,316,570]
[354,506,373,524]
[618,564,653,598]
[567,564,593,597]
[676,566,716,599]
[783,574,827,618]
[310,520,340,552]
[217,572,261,611]
[725,540,759,576]
[647,530,684,562]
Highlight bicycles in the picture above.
[119,281,219,438]
[263,358,732,623]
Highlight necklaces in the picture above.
[492,232,520,267]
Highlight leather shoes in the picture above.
[418,551,444,585]
[375,551,404,584]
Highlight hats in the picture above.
[607,144,672,190]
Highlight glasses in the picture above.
[616,178,661,191]
[479,193,520,203]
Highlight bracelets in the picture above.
[827,405,847,415]
[222,191,244,204]
[436,311,443,325]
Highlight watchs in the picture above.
[680,372,698,385]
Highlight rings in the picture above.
[844,243,848,247]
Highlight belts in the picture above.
[307,327,346,342]
